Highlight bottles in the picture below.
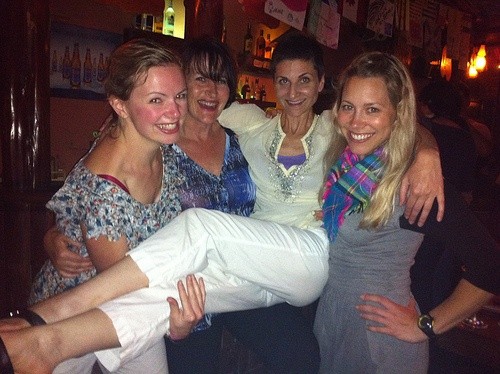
[237,76,268,103]
[50,43,111,89]
[243,24,273,59]
[162,0,175,36]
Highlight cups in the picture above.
[133,13,163,32]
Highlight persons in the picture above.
[265,51,500,373]
[28,38,206,374]
[0,29,444,374]
[44,40,257,374]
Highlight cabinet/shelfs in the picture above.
[48,21,278,108]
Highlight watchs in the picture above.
[418,312,437,339]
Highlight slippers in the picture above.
[8,307,47,326]
[0,336,14,374]
